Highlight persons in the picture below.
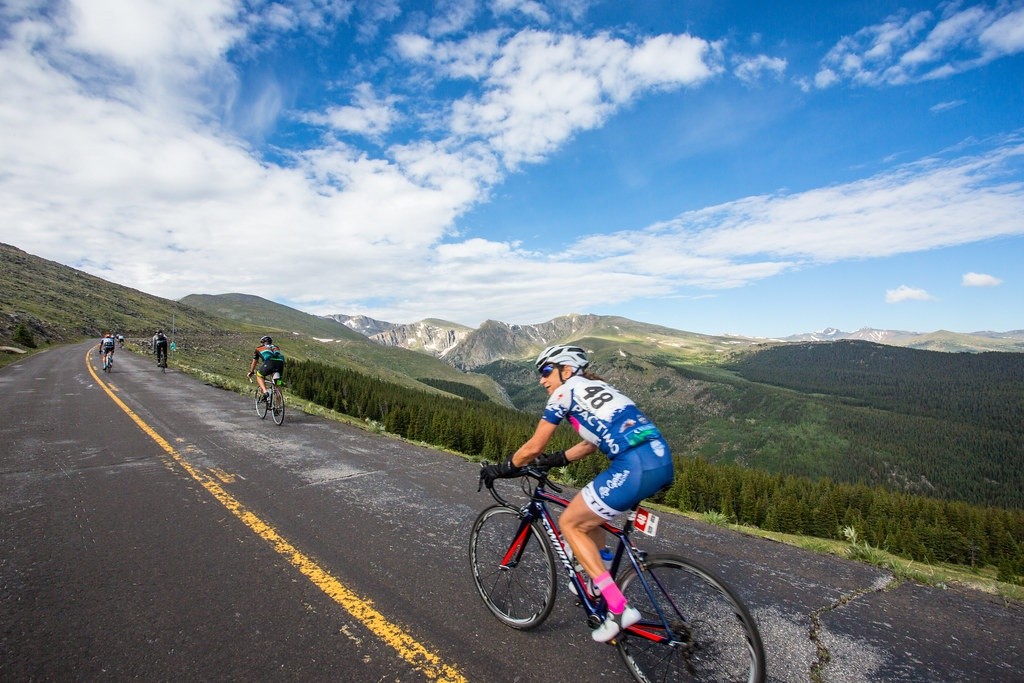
[99,334,115,370]
[247,336,285,416]
[152,329,168,368]
[111,334,124,348]
[481,343,673,642]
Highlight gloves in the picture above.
[247,371,254,378]
[480,461,520,489]
[529,449,572,471]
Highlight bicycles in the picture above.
[153,347,167,375]
[99,349,113,373]
[247,371,286,426]
[469,454,766,683]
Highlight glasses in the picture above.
[542,365,557,378]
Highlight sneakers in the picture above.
[273,405,282,410]
[157,364,161,367]
[165,364,168,368]
[592,600,642,643]
[103,368,107,370]
[259,392,269,403]
[568,581,600,603]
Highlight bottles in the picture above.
[587,545,615,598]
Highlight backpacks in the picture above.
[155,333,167,341]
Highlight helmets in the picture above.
[534,345,590,375]
[155,330,162,333]
[105,334,111,338]
[260,336,273,344]
